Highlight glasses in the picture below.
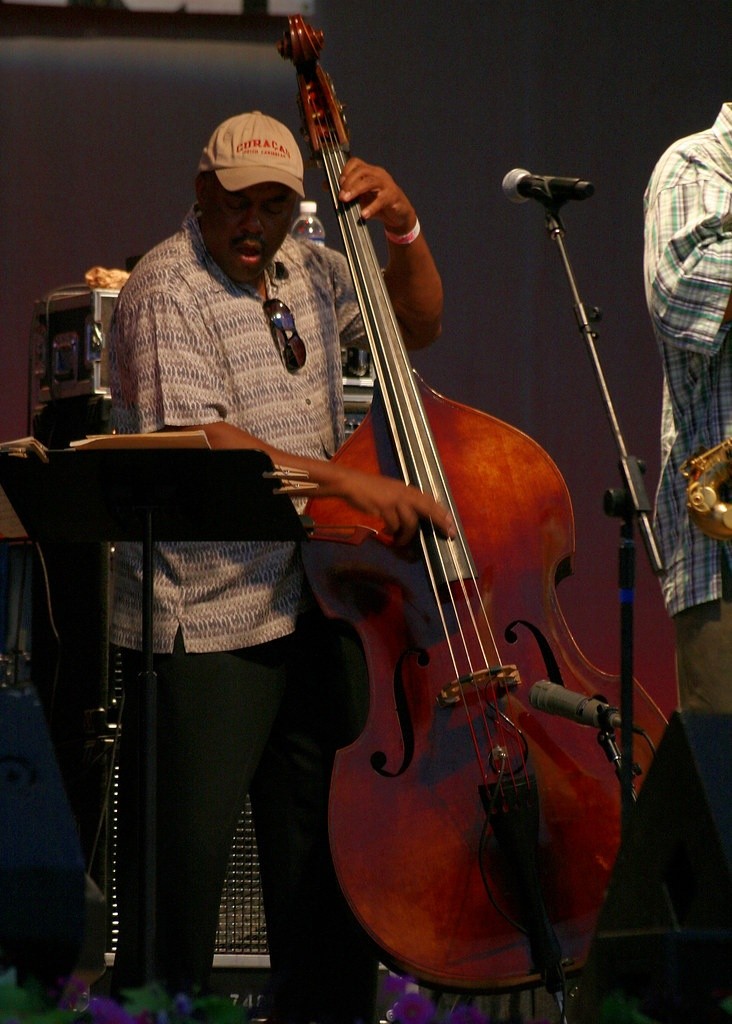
[262,297,306,374]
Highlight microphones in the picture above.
[529,680,644,736]
[502,169,594,203]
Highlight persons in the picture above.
[645,102,731,866]
[106,113,457,1024]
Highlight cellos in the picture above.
[271,12,673,994]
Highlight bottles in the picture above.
[291,201,326,247]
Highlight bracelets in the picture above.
[383,216,420,244]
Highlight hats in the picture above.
[194,109,306,197]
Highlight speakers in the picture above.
[0,681,88,1007]
[212,792,271,970]
[569,708,732,1024]
